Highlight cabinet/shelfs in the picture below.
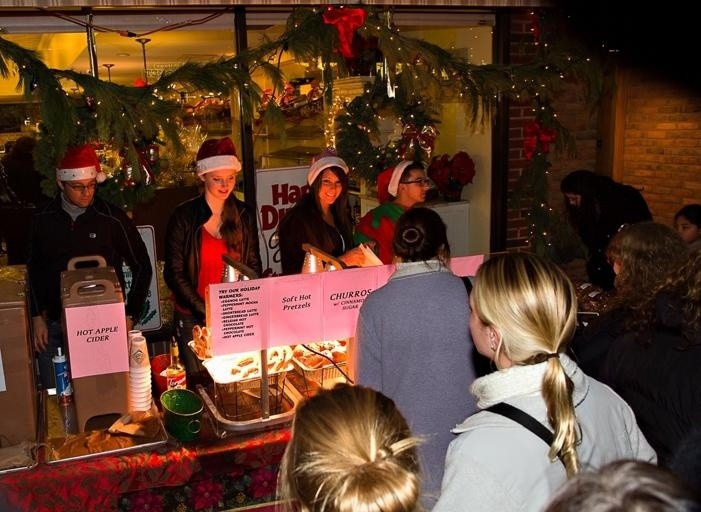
[254,53,333,171]
[329,71,376,200]
[355,194,472,260]
[193,86,238,142]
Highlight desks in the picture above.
[0,383,296,510]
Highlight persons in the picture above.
[164,138,262,385]
[28,144,152,391]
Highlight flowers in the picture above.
[429,151,479,186]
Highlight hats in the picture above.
[307,148,350,185]
[374,159,414,206]
[55,141,107,183]
[197,137,242,180]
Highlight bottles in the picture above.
[52,346,73,407]
[59,406,75,435]
[166,339,187,389]
[127,329,153,411]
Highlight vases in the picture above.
[442,184,465,203]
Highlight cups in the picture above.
[151,354,171,396]
[160,389,204,440]
